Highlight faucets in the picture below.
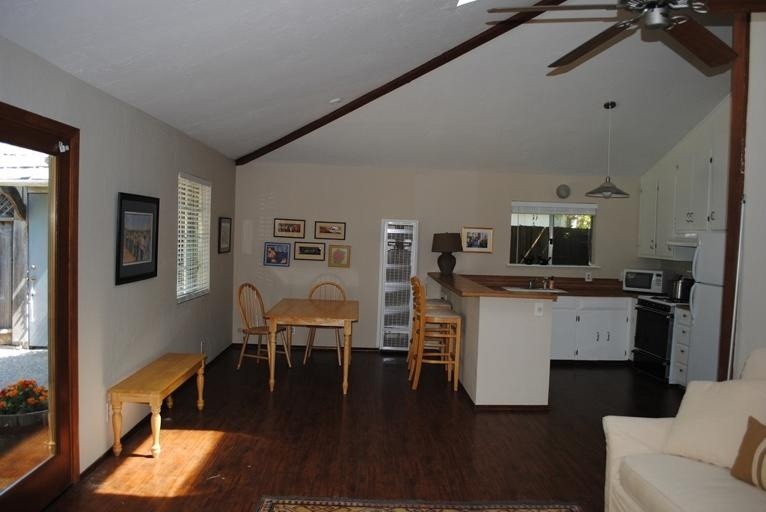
[528,277,535,288]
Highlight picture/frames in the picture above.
[263,218,351,268]
[218,217,232,253]
[462,227,493,254]
[115,191,160,286]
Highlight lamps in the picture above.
[584,101,629,199]
[432,231,463,276]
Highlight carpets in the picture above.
[255,494,585,512]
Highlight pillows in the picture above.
[663,380,766,469]
[731,415,765,492]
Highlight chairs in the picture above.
[407,286,462,392]
[406,276,452,363]
[302,281,347,366]
[237,282,292,369]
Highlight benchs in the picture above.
[107,352,208,458]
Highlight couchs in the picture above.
[602,349,766,512]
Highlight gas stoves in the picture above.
[636,295,690,315]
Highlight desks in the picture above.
[263,298,359,395]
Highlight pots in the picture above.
[673,277,693,302]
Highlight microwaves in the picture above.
[623,269,663,293]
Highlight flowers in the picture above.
[0,378,48,414]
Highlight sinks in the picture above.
[500,284,568,293]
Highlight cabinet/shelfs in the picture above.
[668,307,691,386]
[637,94,731,261]
[551,297,634,361]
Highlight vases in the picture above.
[0,410,48,427]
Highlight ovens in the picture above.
[633,305,675,384]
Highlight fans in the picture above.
[486,0,766,67]
